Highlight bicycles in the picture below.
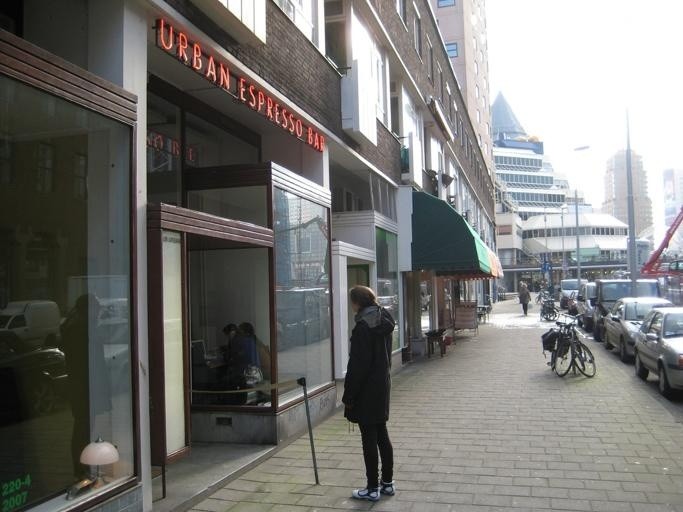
[534,288,560,321]
[547,321,596,378]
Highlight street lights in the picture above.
[543,206,552,287]
[560,206,569,279]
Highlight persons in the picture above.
[519,283,532,315]
[342,285,395,502]
[59,294,113,478]
[222,322,262,383]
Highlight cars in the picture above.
[0,298,131,417]
[377,278,399,325]
[558,279,683,395]
[277,278,330,352]
[420,282,431,310]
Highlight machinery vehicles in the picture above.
[640,204,683,278]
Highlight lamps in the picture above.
[78,435,119,488]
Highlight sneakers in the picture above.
[379,478,395,495]
[352,487,381,502]
